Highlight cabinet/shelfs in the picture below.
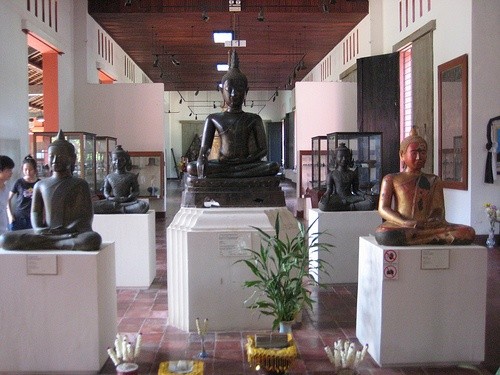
[32,131,117,199]
[127,151,164,200]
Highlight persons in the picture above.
[0,129,101,251]
[187,48,280,178]
[93,144,149,214]
[8,154,44,231]
[318,144,377,212]
[0,155,15,247]
[374,127,475,246]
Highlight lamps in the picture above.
[201,10,209,22]
[153,55,159,67]
[171,55,180,66]
[195,88,199,96]
[189,113,192,116]
[179,99,182,104]
[273,90,279,102]
[288,60,306,85]
[258,8,264,22]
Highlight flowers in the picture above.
[484,202,497,230]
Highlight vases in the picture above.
[487,230,495,248]
[280,319,295,335]
